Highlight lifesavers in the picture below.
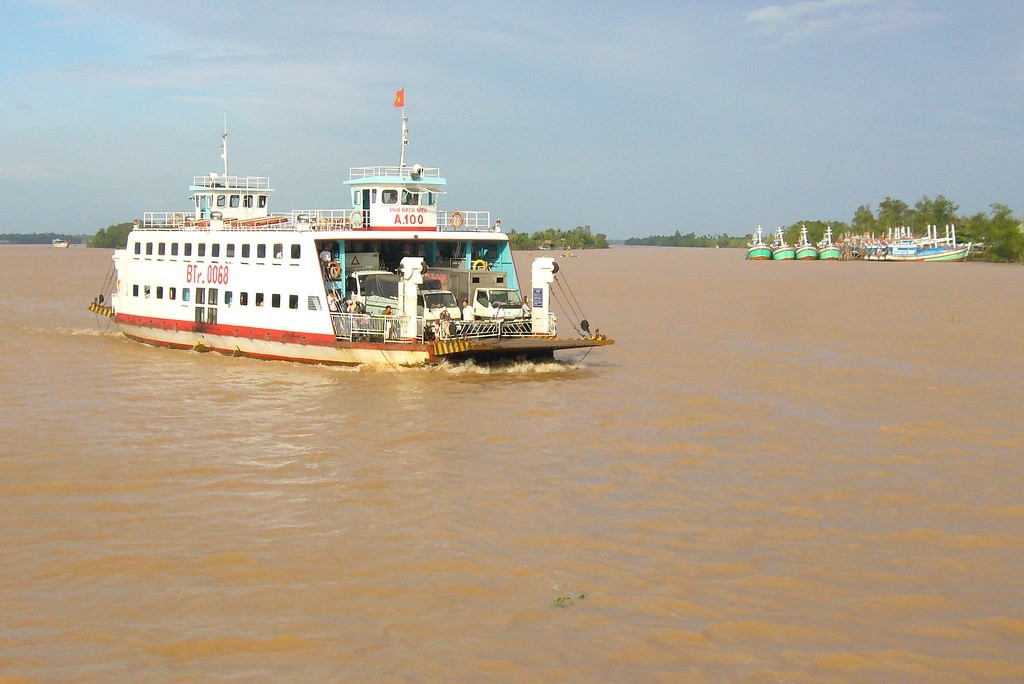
[327,262,340,278]
[473,259,486,271]
[496,219,501,232]
[171,213,183,226]
[116,278,122,290]
[350,211,364,227]
[450,212,464,228]
[435,320,440,343]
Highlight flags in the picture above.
[394,89,403,107]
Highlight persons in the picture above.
[523,299,531,317]
[490,303,505,324]
[440,307,450,336]
[387,192,418,205]
[319,244,394,340]
[462,300,475,336]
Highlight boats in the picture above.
[744,225,773,261]
[86,86,619,368]
[798,225,820,261]
[817,226,842,260]
[770,226,798,262]
[53,239,71,248]
[837,223,974,263]
